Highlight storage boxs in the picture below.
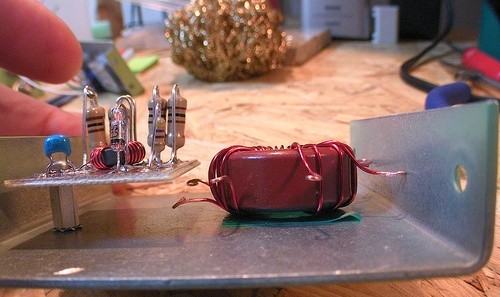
[280,0,370,40]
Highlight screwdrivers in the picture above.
[442,37,500,82]
